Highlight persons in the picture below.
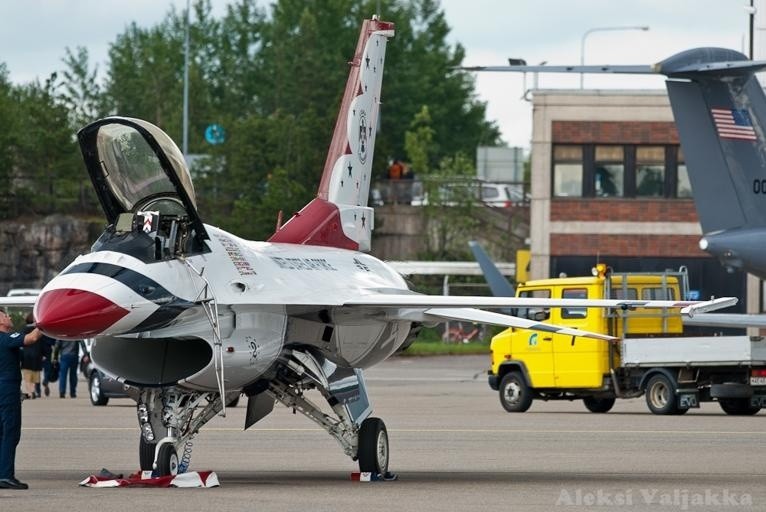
[462,321,482,344]
[0,305,43,489]
[20,312,89,401]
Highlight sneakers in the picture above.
[60,392,75,397]
[21,381,49,400]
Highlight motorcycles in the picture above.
[441,320,488,346]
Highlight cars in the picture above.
[79,351,133,404]
[414,176,529,207]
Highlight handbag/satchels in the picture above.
[80,353,90,370]
[49,362,59,381]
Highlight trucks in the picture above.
[487,264,766,417]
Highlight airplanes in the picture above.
[30,13,741,477]
[450,46,766,283]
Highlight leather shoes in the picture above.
[0,479,28,489]
